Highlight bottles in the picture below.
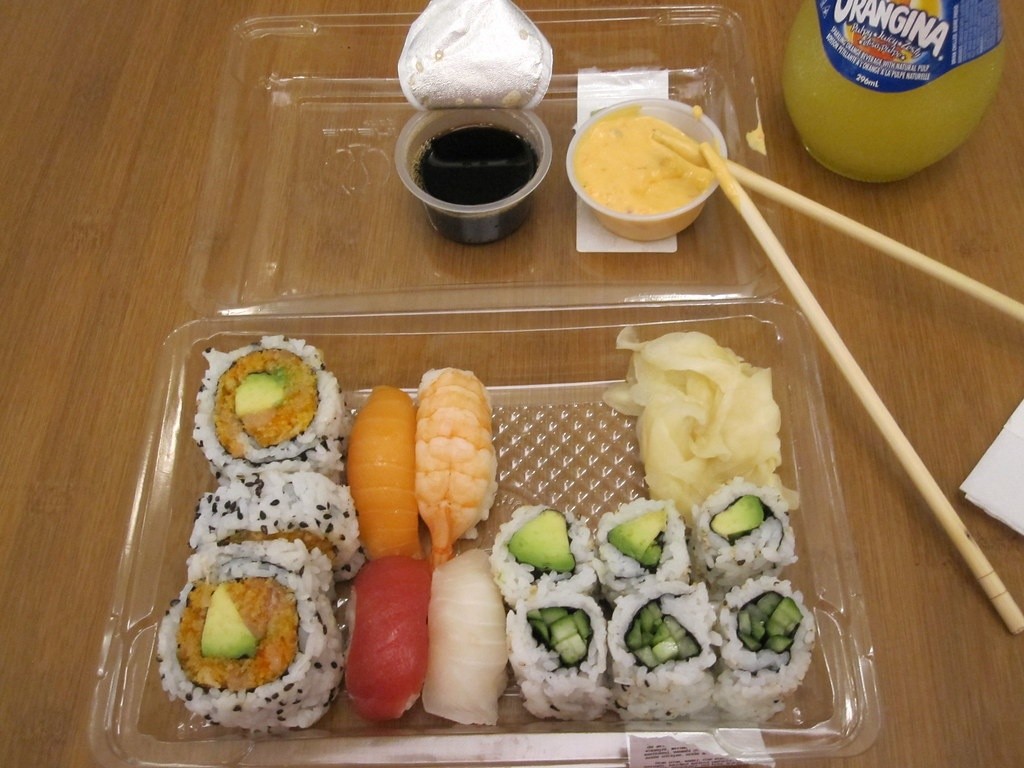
[782,0,1004,183]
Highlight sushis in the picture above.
[156,334,819,733]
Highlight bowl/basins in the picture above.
[566,98,728,241]
[394,110,552,244]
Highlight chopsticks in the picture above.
[651,130,1024,635]
